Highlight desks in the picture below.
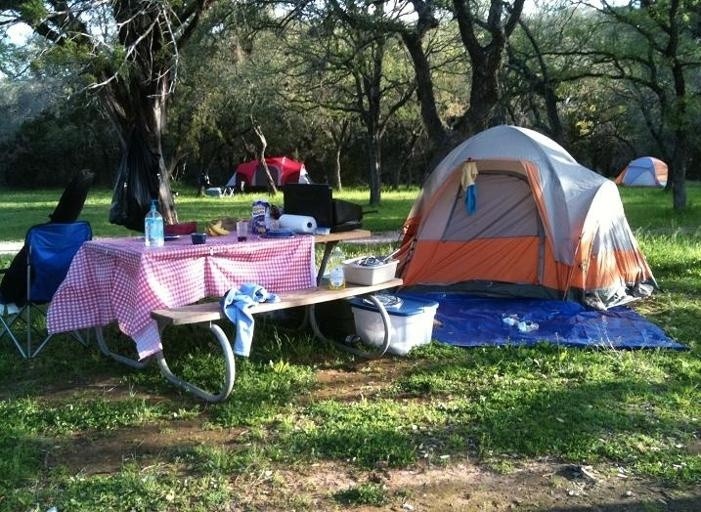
[82,226,394,400]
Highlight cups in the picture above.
[236,222,248,243]
[192,232,206,244]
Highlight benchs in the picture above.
[150,274,405,402]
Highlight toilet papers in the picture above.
[278,214,317,233]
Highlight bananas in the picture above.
[206,220,230,236]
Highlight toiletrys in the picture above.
[144,200,164,246]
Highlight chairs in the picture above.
[1,221,94,362]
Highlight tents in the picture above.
[224,155,311,190]
[393,124,665,311]
[614,157,670,187]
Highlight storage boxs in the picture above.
[347,293,441,357]
[341,253,401,286]
